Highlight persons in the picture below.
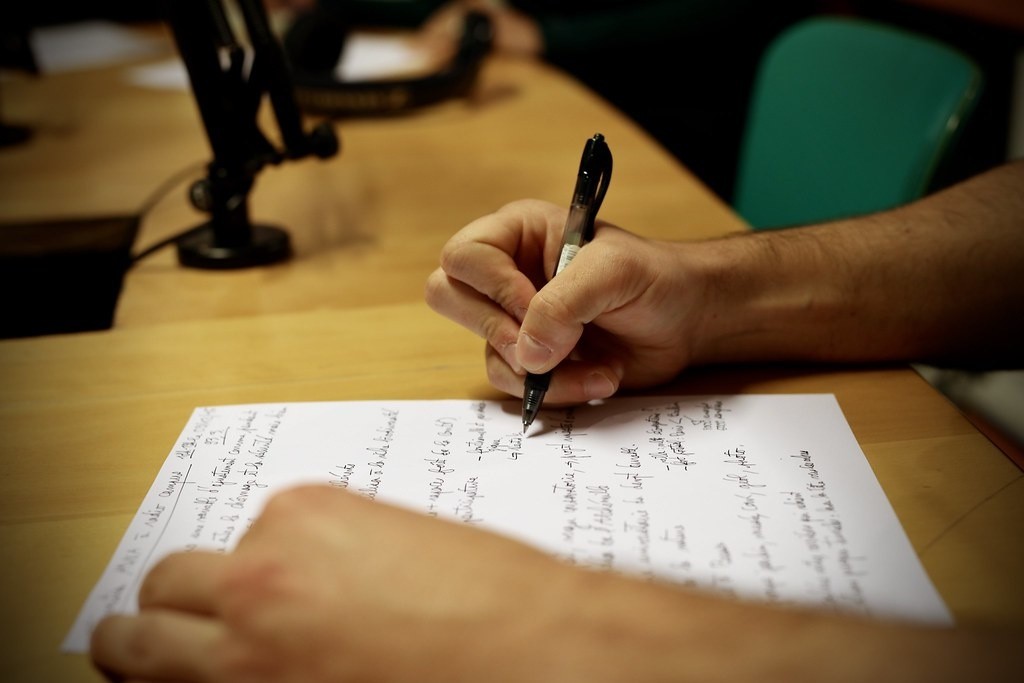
[90,157,1024,683]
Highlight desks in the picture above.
[0,0,1024,683]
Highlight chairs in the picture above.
[733,16,985,230]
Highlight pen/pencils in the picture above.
[521,132,615,435]
